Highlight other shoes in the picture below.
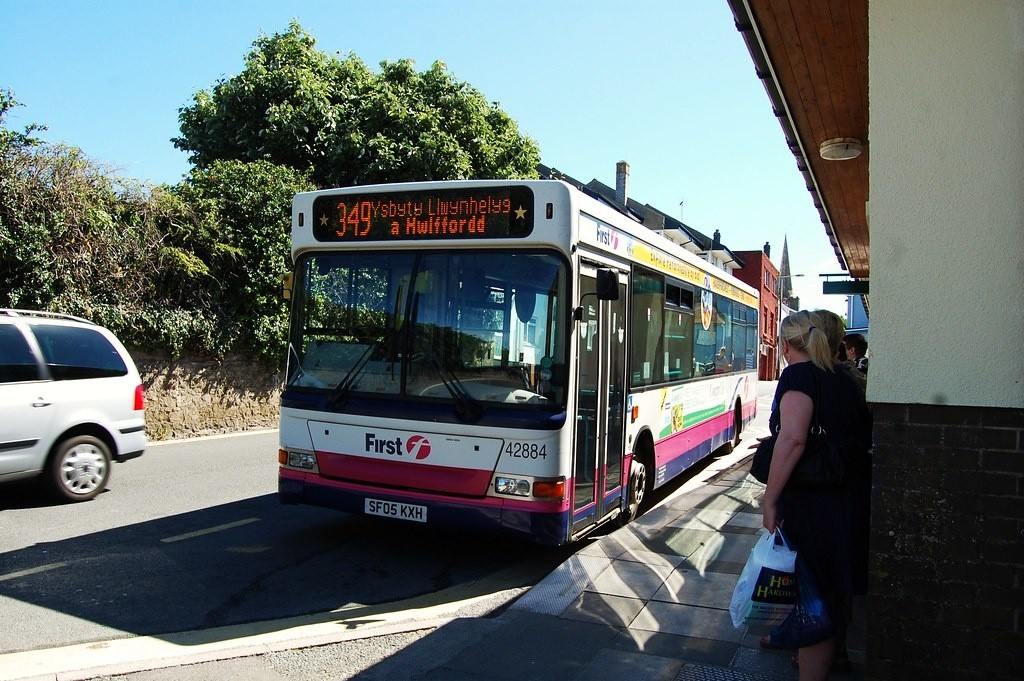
[760,635,776,648]
[791,654,852,672]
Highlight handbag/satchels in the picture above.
[749,363,829,492]
[728,526,796,635]
[770,530,832,648]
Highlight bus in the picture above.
[279,180,761,548]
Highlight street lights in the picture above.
[775,274,805,381]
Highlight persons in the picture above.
[712,347,731,374]
[754,309,874,681]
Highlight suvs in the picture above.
[0,308,147,503]
